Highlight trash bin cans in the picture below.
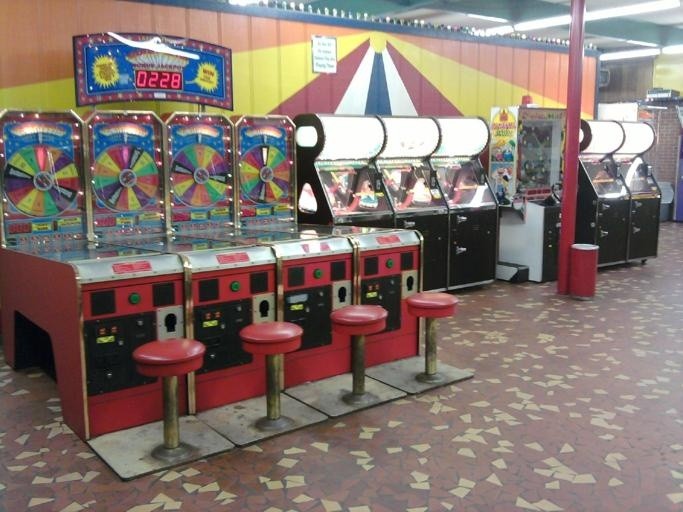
[570,243,599,301]
[656,181,674,223]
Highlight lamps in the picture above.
[460,1,682,63]
[220,0,283,10]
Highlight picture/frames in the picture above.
[311,34,338,76]
